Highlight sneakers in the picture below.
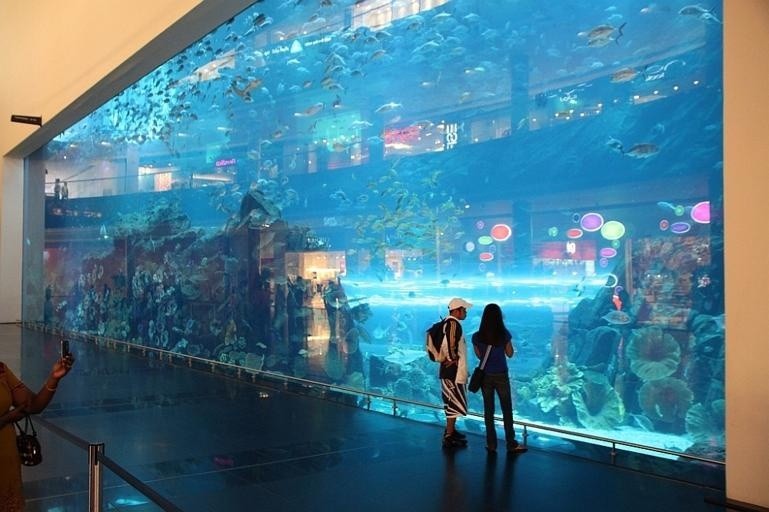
[485,442,496,451]
[440,447,468,455]
[506,440,528,453]
[441,429,468,446]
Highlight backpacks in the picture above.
[426,319,445,362]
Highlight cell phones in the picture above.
[59,340,69,360]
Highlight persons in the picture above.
[439,298,468,448]
[0,352,75,512]
[54,179,68,200]
[472,304,528,453]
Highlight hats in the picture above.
[447,297,472,310]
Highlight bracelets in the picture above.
[44,381,58,391]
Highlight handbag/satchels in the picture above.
[468,368,485,393]
[17,435,42,466]
